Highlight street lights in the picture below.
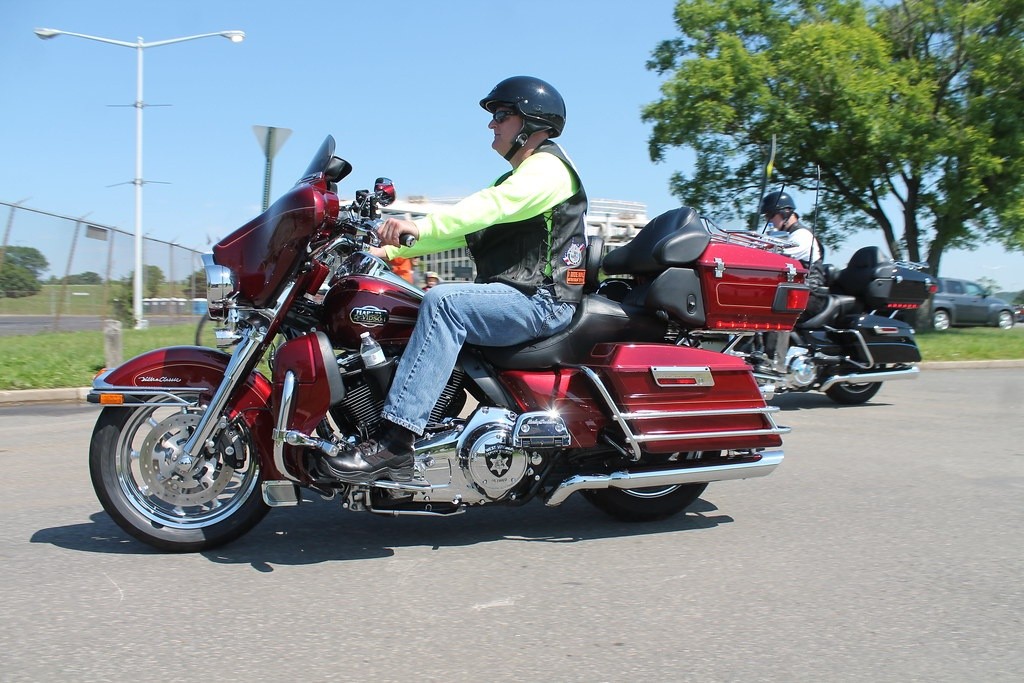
[34,29,247,330]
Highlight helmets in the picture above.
[760,192,796,213]
[479,76,566,137]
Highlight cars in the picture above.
[930,276,1024,334]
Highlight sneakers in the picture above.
[320,429,415,484]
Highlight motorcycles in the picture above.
[716,237,935,408]
[77,126,794,550]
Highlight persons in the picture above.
[753,192,825,377]
[320,76,588,482]
[422,272,439,291]
[387,257,420,285]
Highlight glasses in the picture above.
[493,110,524,122]
[765,211,782,217]
